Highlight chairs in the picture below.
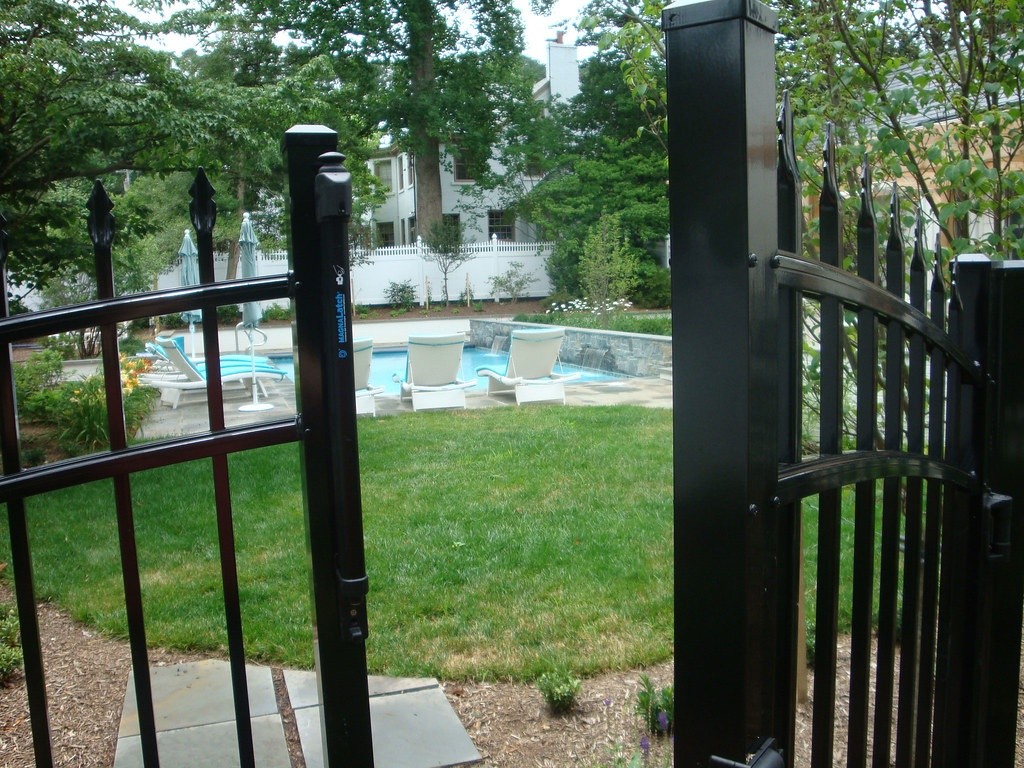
[144,337,287,398]
[353,339,382,416]
[402,332,476,411]
[475,326,566,405]
